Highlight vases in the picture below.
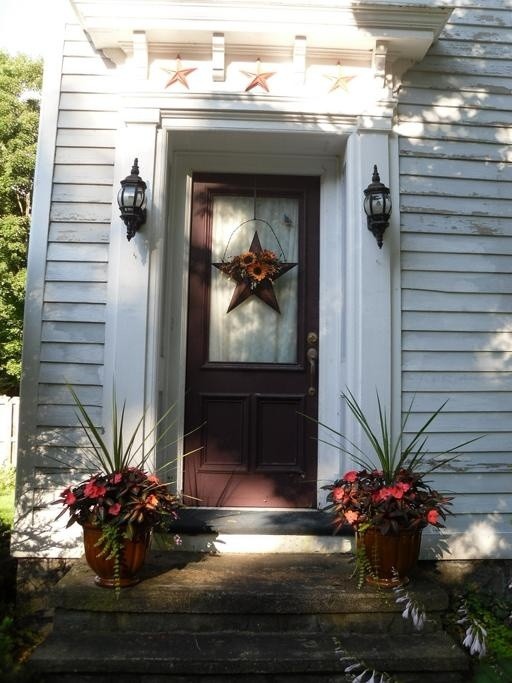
[352,526,421,588]
[81,522,154,593]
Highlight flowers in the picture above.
[289,371,489,589]
[27,364,206,599]
[221,251,285,292]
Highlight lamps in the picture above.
[116,157,148,243]
[362,165,394,249]
